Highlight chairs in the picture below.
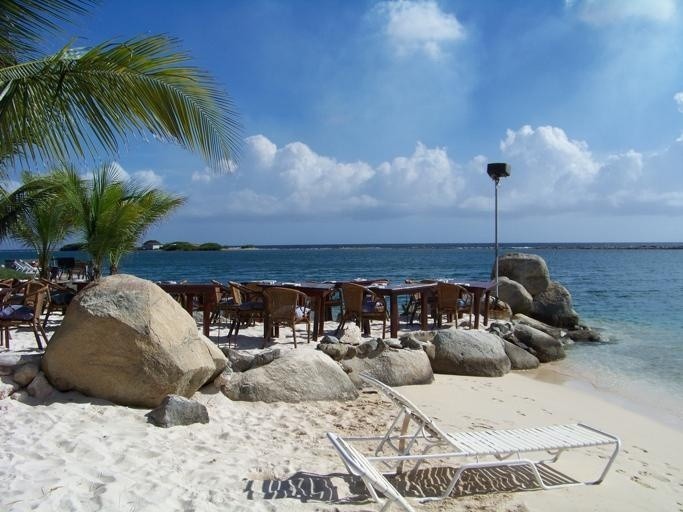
[326,432,415,512]
[337,372,621,505]
